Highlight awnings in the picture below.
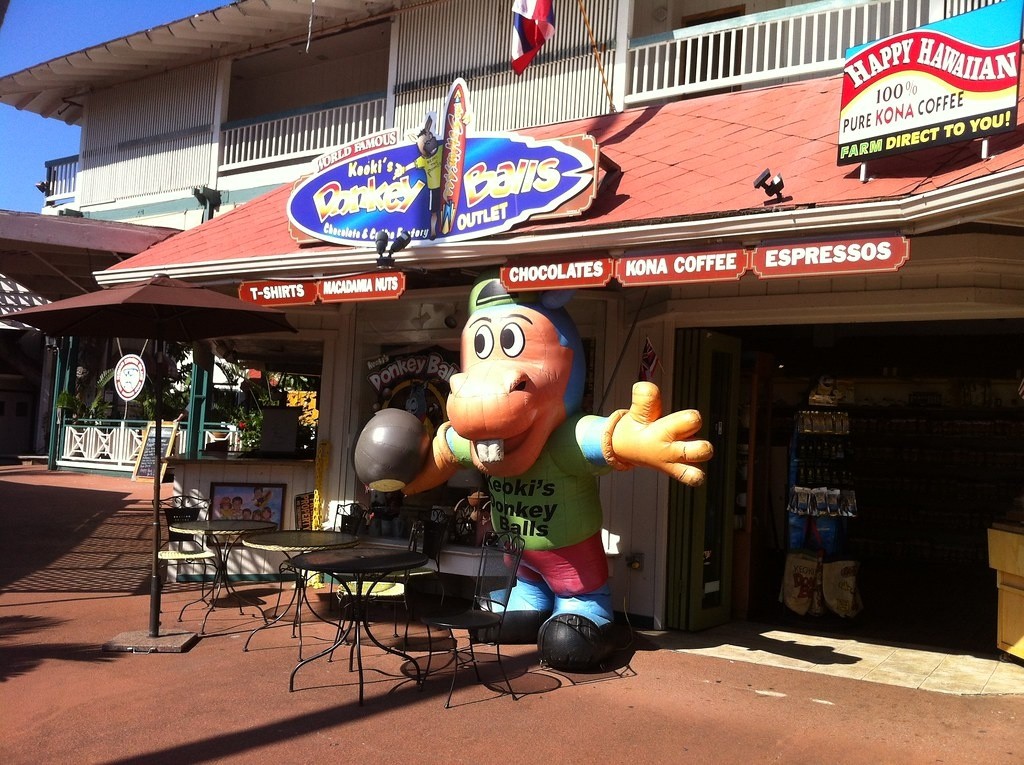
[1,208,190,304]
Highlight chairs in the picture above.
[418,530,525,709]
[272,501,473,661]
[149,495,216,610]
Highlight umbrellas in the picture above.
[1,267,303,634]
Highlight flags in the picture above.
[509,0,558,76]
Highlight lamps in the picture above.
[388,230,412,257]
[192,185,222,212]
[754,169,786,200]
[375,231,389,259]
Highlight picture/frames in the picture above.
[206,483,287,546]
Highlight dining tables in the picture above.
[242,531,358,652]
[285,544,428,707]
[168,520,276,636]
